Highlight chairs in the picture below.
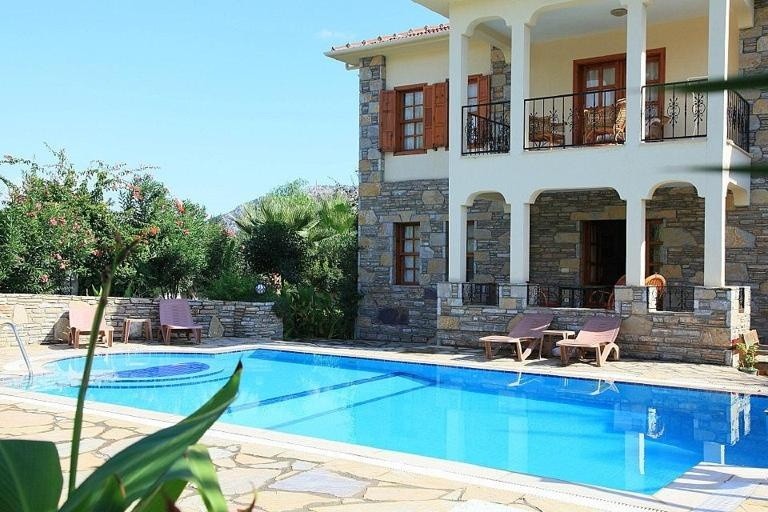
[158,299,202,345]
[529,98,626,146]
[479,309,555,362]
[68,300,114,350]
[556,318,620,367]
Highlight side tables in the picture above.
[539,329,577,360]
[121,317,153,344]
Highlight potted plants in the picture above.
[735,341,759,375]
